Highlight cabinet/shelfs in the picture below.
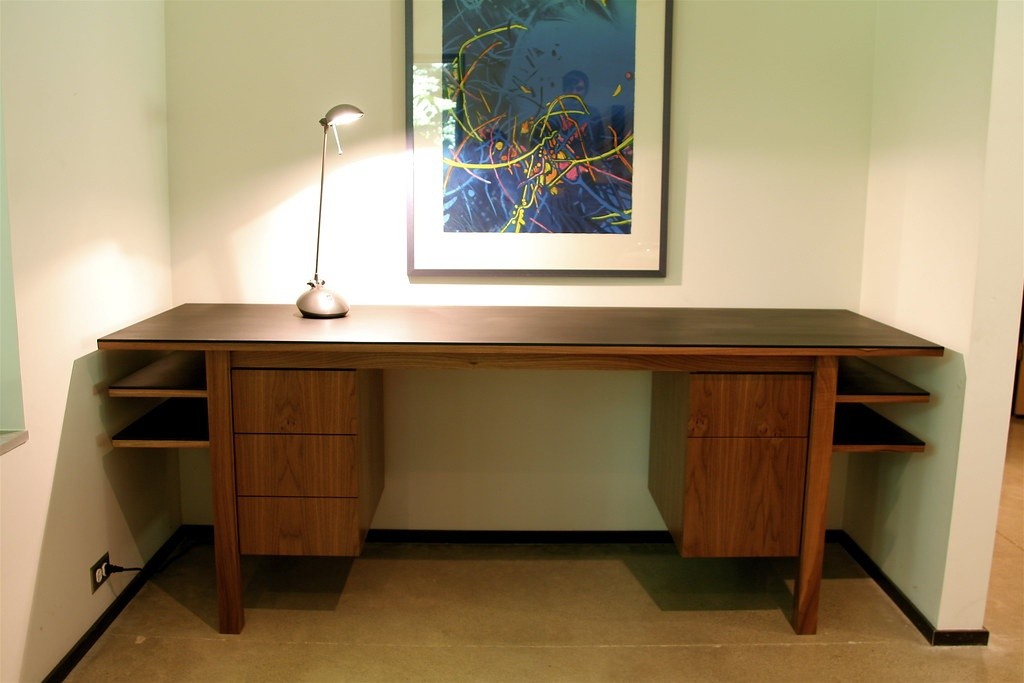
[96,301,943,635]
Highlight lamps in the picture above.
[296,102,364,318]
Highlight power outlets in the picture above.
[90,552,111,595]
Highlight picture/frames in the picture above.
[405,0,674,279]
